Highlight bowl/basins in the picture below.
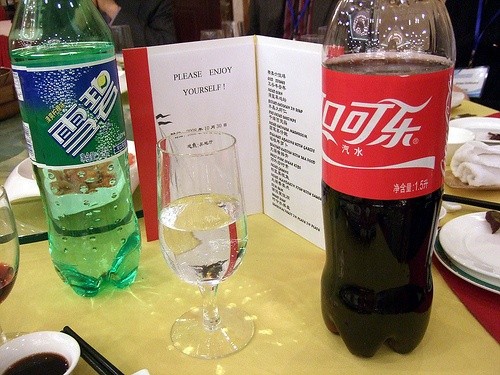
[446,125,475,168]
[0,330,81,375]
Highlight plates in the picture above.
[449,116,500,144]
[0,140,140,204]
[439,211,500,280]
[433,229,500,295]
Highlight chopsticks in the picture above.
[442,194,500,210]
[59,326,124,375]
[18,209,143,245]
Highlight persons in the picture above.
[66,0,176,48]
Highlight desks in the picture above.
[0,53,499,375]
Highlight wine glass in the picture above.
[1,184,28,348]
[156,130,256,360]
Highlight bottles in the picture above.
[320,0,456,358]
[8,0,142,296]
